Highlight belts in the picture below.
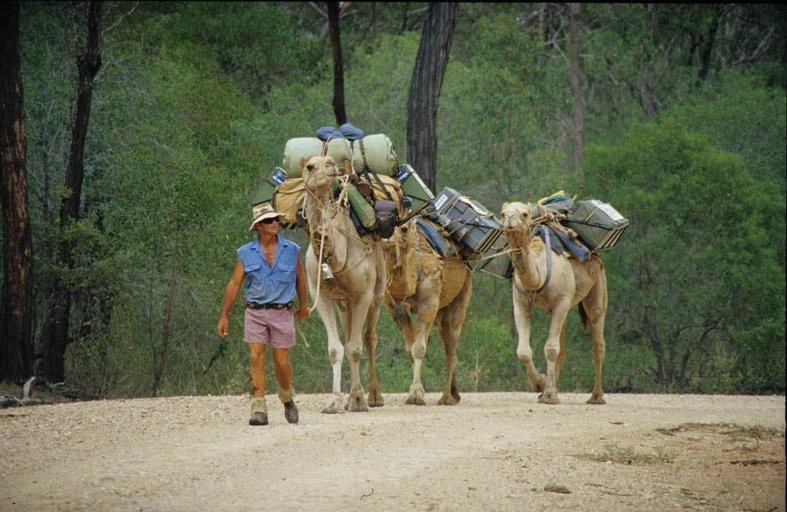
[247,301,290,311]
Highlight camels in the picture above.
[502,199,611,406]
[381,214,474,408]
[300,152,388,415]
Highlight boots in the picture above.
[276,386,300,425]
[248,398,269,426]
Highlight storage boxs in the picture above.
[250,167,284,207]
[394,162,627,279]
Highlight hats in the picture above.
[246,201,287,232]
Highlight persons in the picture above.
[217,199,311,425]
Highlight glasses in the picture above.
[262,218,281,224]
